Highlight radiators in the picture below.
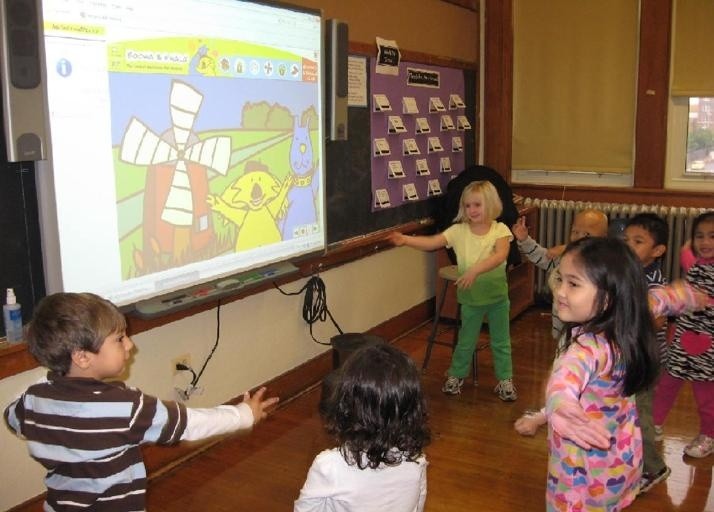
[517,197,714,304]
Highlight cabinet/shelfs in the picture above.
[434,205,538,324]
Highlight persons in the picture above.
[4,293,280,512]
[384,180,518,402]
[651,211,714,458]
[513,390,672,496]
[547,237,713,512]
[512,210,607,338]
[294,345,432,512]
[622,214,671,360]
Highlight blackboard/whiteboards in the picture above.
[1,40,480,379]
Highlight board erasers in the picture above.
[216,279,241,291]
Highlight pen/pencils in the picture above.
[240,270,281,283]
[161,286,212,304]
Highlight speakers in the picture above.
[0,0,49,163]
[327,17,349,143]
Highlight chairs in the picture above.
[420,166,519,385]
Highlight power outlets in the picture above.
[170,355,191,375]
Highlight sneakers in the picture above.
[493,379,519,401]
[441,376,464,394]
[654,425,714,458]
[637,466,671,493]
